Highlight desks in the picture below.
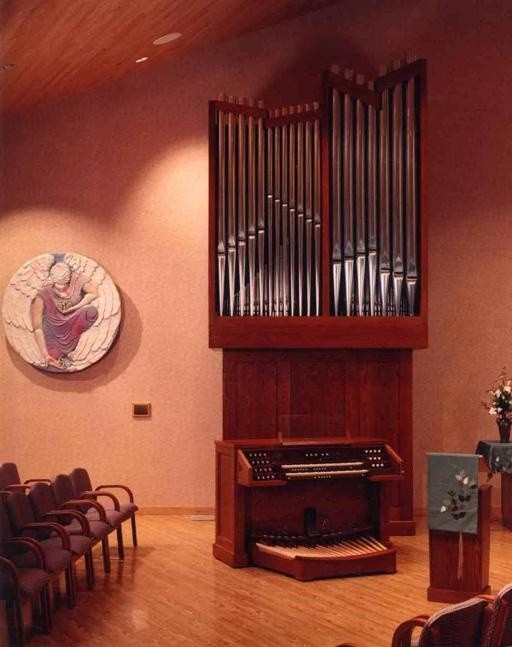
[475,440,512,531]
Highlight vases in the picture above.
[496,418,512,443]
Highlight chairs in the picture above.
[337,583,512,647]
[0,463,139,647]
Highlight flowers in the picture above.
[483,366,512,419]
